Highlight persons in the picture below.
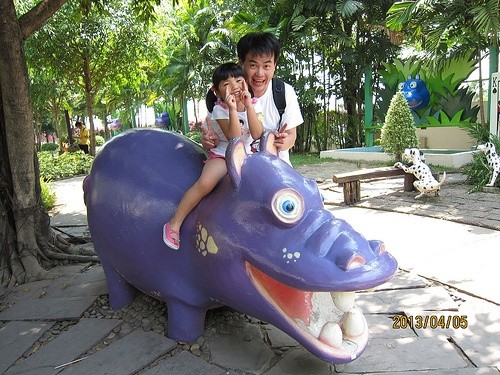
[72,121,89,154]
[163,63,264,251]
[200,32,304,168]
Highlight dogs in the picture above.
[476,141,499,188]
[393,147,447,200]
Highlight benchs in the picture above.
[331,164,415,204]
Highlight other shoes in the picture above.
[162,221,180,250]
